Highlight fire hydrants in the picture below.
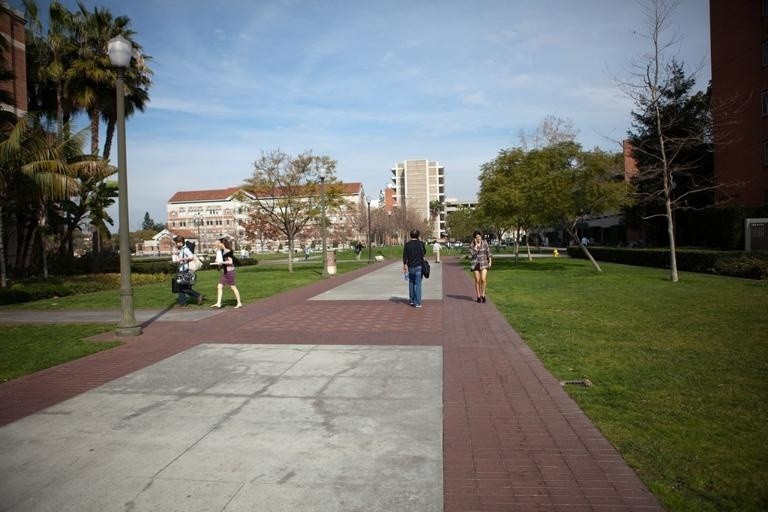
[552,249,559,257]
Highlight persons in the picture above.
[402,228,426,308]
[354,241,364,260]
[209,237,243,310]
[239,246,250,259]
[432,240,442,263]
[303,244,312,261]
[169,234,203,308]
[468,230,492,304]
[580,234,587,246]
[589,236,595,245]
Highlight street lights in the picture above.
[106,29,142,342]
[364,195,374,264]
[385,211,392,247]
[316,165,329,276]
[352,222,362,260]
[194,211,203,254]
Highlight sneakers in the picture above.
[409,301,422,307]
[171,293,243,309]
[476,296,486,303]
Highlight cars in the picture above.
[503,238,513,246]
[445,240,463,248]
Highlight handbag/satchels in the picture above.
[186,241,196,253]
[171,279,192,293]
[422,260,432,278]
[177,271,195,285]
[187,254,204,273]
